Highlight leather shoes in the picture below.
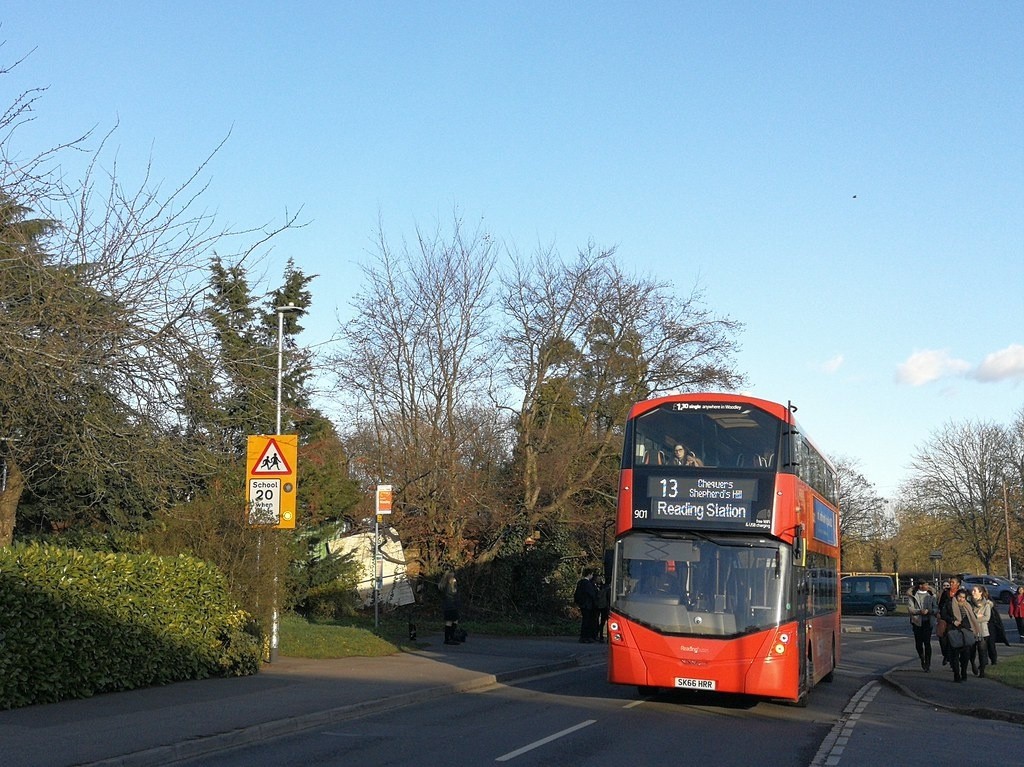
[580,638,594,644]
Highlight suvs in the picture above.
[962,575,1019,604]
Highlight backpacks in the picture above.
[574,580,589,604]
[598,587,610,609]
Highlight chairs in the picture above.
[737,451,774,467]
[642,449,666,465]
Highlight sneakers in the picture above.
[1021,635,1024,638]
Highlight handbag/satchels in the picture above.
[452,627,468,643]
[948,625,975,648]
[936,618,947,637]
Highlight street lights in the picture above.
[244,307,310,665]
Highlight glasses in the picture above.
[674,449,682,453]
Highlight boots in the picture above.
[444,623,459,644]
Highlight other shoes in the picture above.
[978,669,985,678]
[942,656,947,665]
[595,636,604,643]
[991,659,997,665]
[961,669,967,681]
[972,662,978,674]
[954,672,961,681]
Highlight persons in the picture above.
[572,566,612,646]
[908,578,1024,683]
[661,444,706,467]
[437,570,461,646]
[624,570,638,594]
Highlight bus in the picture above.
[603,392,841,708]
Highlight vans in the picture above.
[841,576,897,616]
[898,572,972,601]
